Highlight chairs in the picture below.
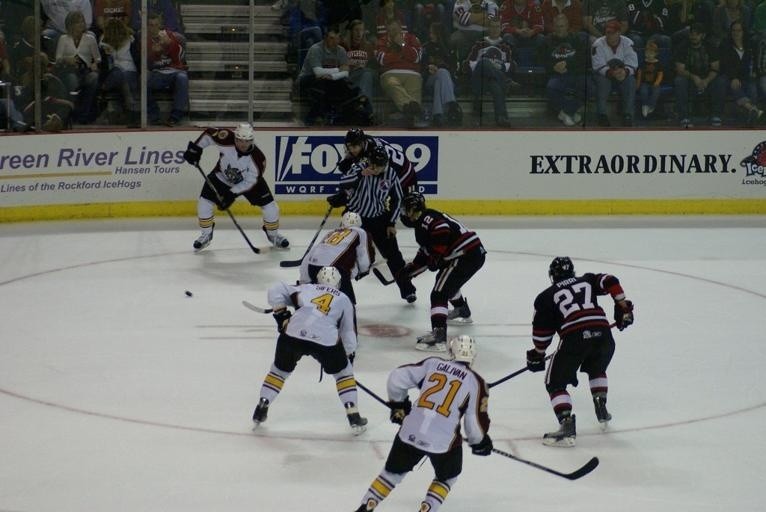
[282,0,765,130]
[0,1,190,128]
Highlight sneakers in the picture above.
[446,302,472,318]
[252,404,268,424]
[557,104,764,129]
[403,293,418,303]
[192,223,216,248]
[416,326,450,344]
[345,409,368,427]
[593,399,612,423]
[543,413,576,441]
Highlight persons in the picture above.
[183,119,292,255]
[339,144,418,309]
[354,335,493,511]
[298,211,376,340]
[250,268,368,436]
[527,255,634,449]
[284,0,765,128]
[0,0,191,132]
[400,191,489,353]
[325,127,418,208]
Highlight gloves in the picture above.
[396,260,418,281]
[327,189,348,208]
[613,300,635,331]
[526,348,547,373]
[270,307,293,332]
[469,435,493,457]
[183,140,203,165]
[217,190,236,211]
[390,396,411,425]
[427,250,441,271]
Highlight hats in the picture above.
[605,20,621,33]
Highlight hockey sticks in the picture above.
[279,204,333,267]
[242,301,273,313]
[194,163,261,254]
[373,265,430,285]
[464,437,598,480]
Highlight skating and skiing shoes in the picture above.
[262,225,290,248]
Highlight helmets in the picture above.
[448,334,479,366]
[233,122,255,141]
[398,191,424,229]
[340,211,363,228]
[345,126,366,154]
[548,255,576,282]
[315,266,343,290]
[368,144,388,176]
